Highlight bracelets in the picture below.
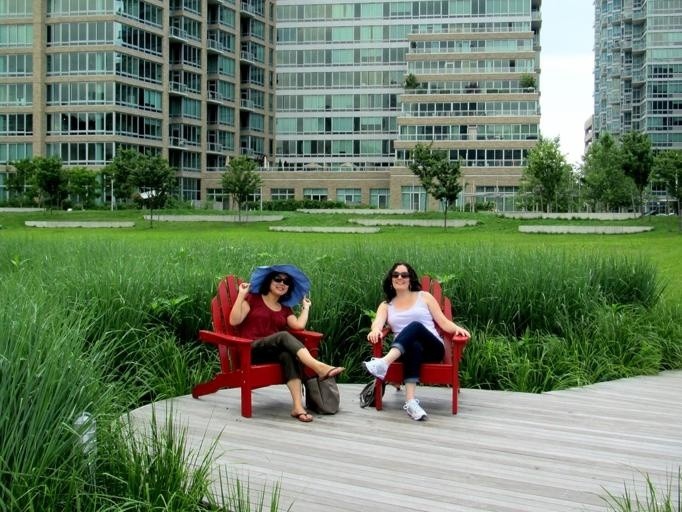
[301,306,310,310]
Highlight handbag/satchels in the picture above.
[306,374,341,416]
[358,377,388,409]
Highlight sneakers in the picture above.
[364,357,390,381]
[402,398,428,422]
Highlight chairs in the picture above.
[193,275,326,419]
[365,277,468,414]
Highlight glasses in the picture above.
[390,270,411,279]
[270,275,292,287]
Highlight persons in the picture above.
[361,262,472,420]
[228,263,346,422]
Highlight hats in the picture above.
[248,264,313,309]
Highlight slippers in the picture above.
[290,411,314,423]
[317,366,346,383]
